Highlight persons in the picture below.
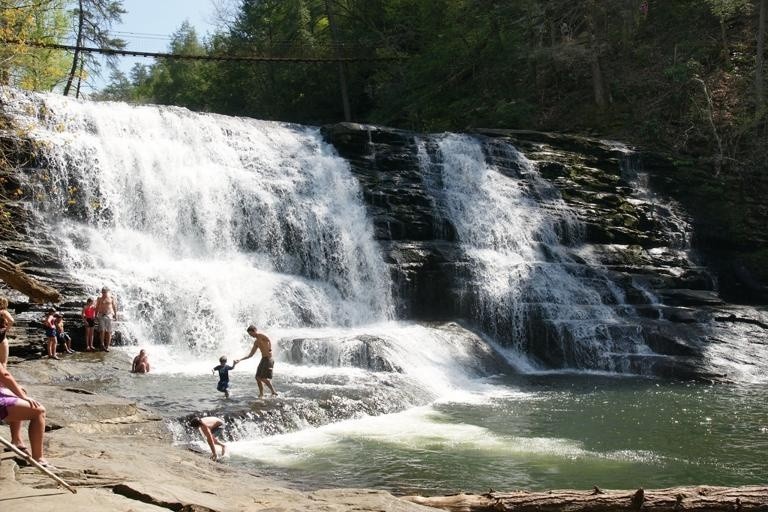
[235,324,279,396]
[55,315,75,354]
[0,295,14,370]
[81,297,96,350]
[46,307,60,360]
[131,349,150,373]
[0,364,54,467]
[95,286,117,352]
[189,416,227,458]
[212,355,236,399]
